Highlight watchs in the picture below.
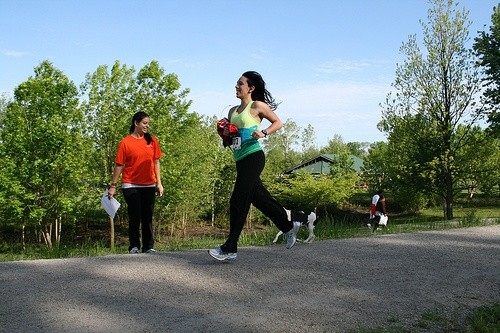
[261,129,267,137]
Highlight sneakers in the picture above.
[367,223,371,230]
[144,248,157,253]
[130,246,139,253]
[286,222,301,249]
[208,246,237,261]
[377,228,382,231]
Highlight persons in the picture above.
[363,182,386,233]
[208,71,301,261]
[107,111,164,254]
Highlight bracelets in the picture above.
[110,182,117,188]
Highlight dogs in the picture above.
[272,205,319,244]
[367,215,390,235]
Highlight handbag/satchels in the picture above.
[379,215,388,225]
